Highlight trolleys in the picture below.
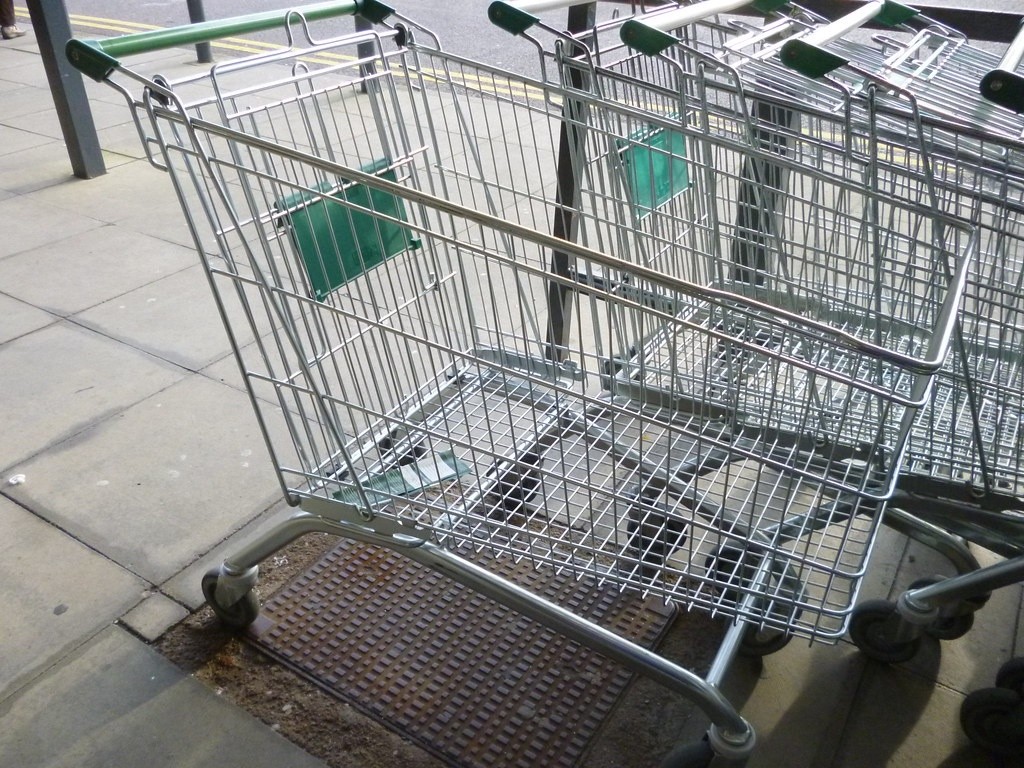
[66,0,977,768]
[487,0,1024,760]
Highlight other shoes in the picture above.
[2,24,26,39]
